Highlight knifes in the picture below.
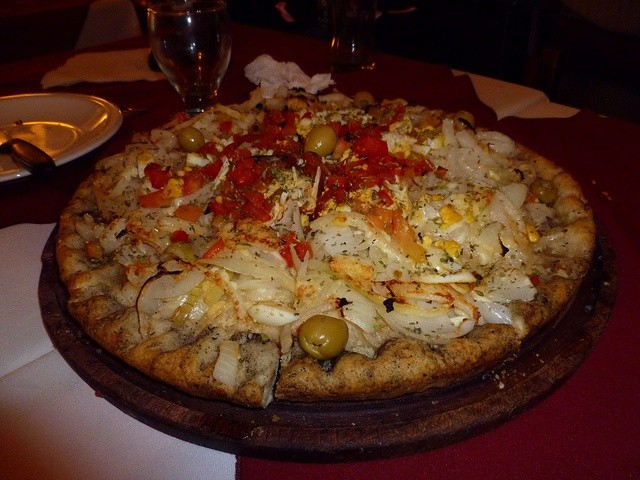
[0,126,72,200]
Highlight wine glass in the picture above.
[325,0,379,79]
[150,1,232,119]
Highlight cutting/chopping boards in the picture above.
[42,189,614,466]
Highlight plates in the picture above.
[0,93,123,184]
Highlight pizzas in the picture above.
[56,85,597,410]
